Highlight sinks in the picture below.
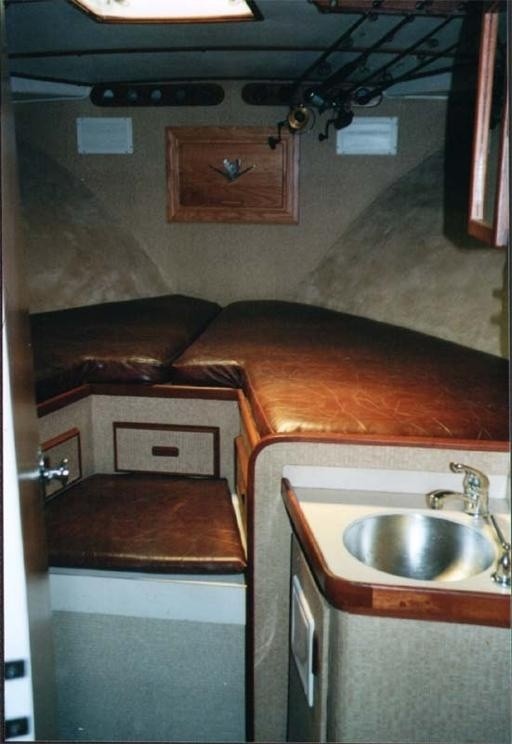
[346,513,495,582]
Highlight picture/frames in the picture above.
[165,124,300,226]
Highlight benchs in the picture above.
[20,295,511,475]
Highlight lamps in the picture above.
[70,0,266,26]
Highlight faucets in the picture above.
[427,463,490,516]
